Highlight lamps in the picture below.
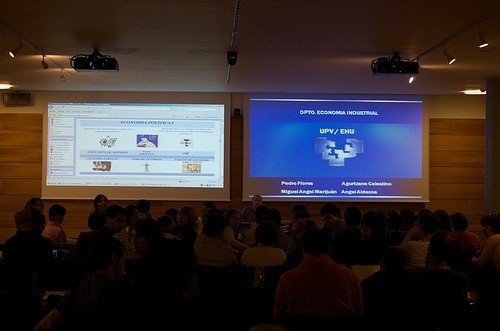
[8,37,23,57]
[478,24,488,48]
[442,44,456,65]
[40,54,49,70]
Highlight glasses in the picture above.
[252,196,262,199]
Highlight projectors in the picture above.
[372,59,419,75]
[73,57,119,73]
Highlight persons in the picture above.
[0,194,500,331]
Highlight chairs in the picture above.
[195,263,286,310]
